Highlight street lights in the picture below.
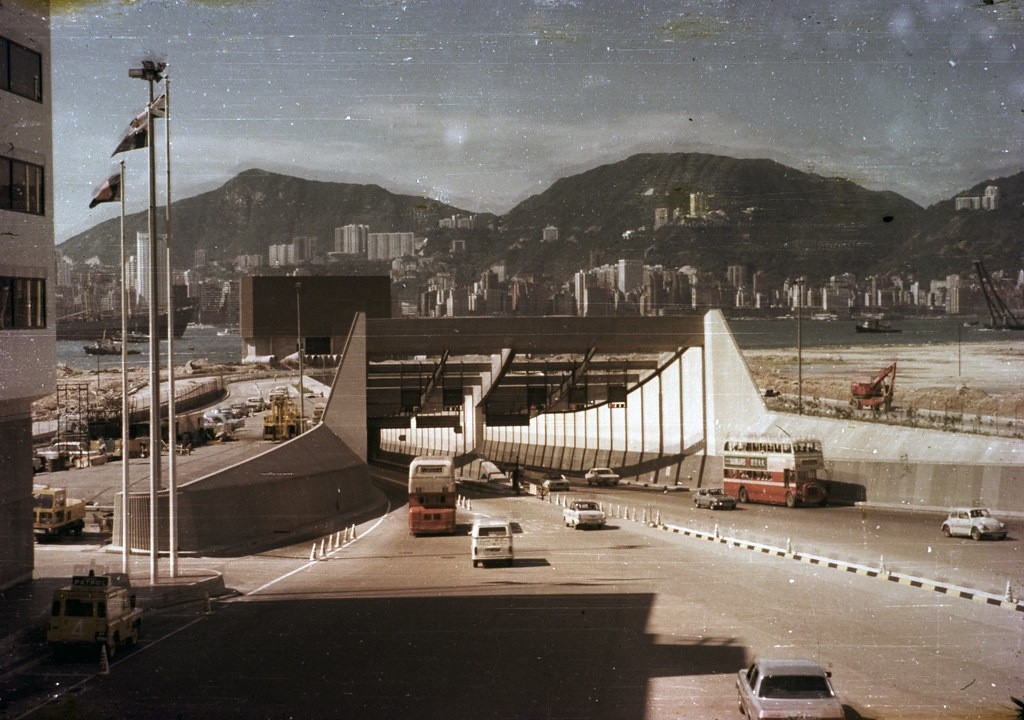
[126,49,167,586]
[795,275,806,416]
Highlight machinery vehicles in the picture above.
[849,361,897,413]
[263,385,309,441]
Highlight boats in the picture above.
[775,310,904,334]
[55,299,196,356]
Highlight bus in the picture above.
[721,436,827,508]
[408,455,458,537]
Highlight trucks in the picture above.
[32,483,86,544]
[45,560,143,664]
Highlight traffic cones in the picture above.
[456,493,472,512]
[544,491,662,527]
[1004,580,1015,604]
[714,523,721,539]
[95,644,113,680]
[880,554,888,575]
[309,523,357,561]
[786,538,792,553]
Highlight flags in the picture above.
[89,173,122,209]
[111,91,166,157]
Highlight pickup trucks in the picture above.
[37,441,100,470]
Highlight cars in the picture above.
[736,658,847,720]
[540,473,570,492]
[691,487,738,510]
[585,467,620,486]
[563,500,607,531]
[203,397,265,433]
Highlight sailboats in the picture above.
[961,260,1024,333]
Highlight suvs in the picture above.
[940,505,1009,541]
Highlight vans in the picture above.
[468,518,515,567]
